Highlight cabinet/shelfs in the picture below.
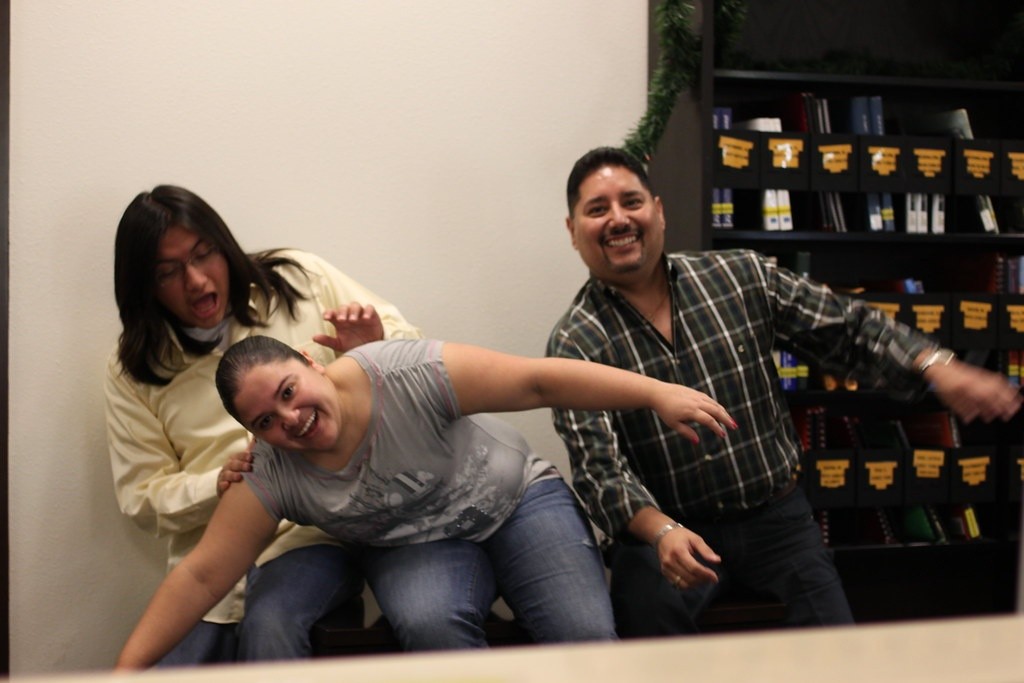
[648,0,1024,617]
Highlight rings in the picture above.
[674,575,681,584]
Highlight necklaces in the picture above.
[628,288,670,322]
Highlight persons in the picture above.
[546,146,1022,641]
[110,333,738,673]
[107,185,385,666]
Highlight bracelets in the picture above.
[653,522,684,548]
[918,348,953,372]
